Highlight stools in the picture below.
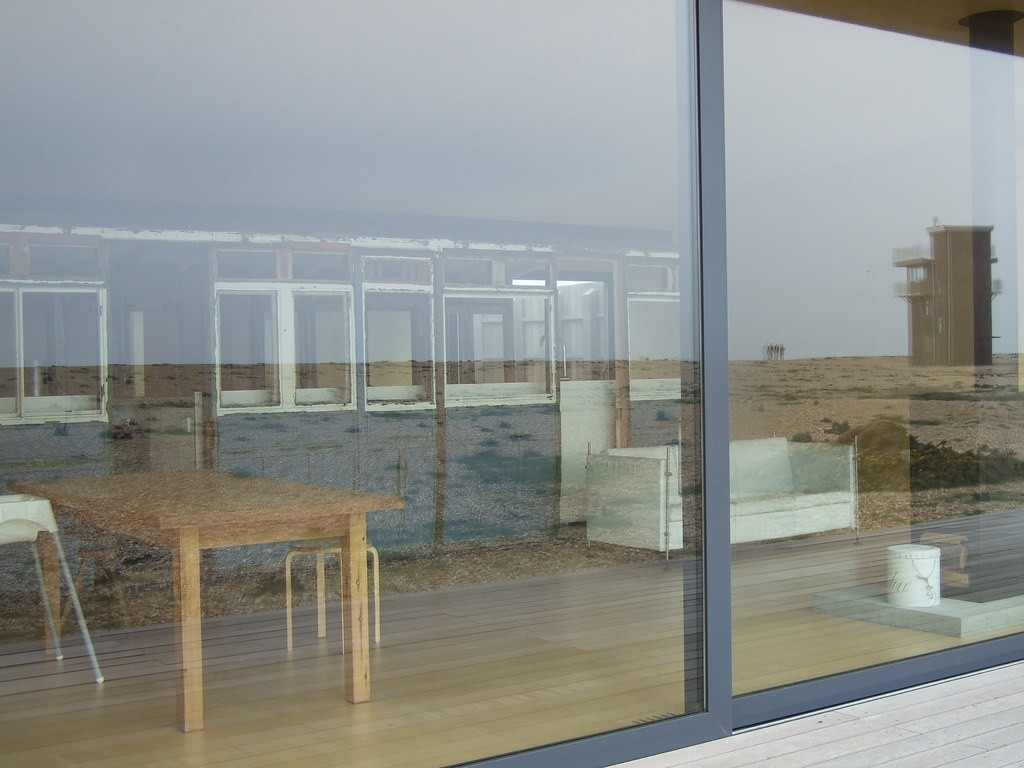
[284,541,381,655]
[0,494,105,684]
[885,542,940,607]
[59,525,168,635]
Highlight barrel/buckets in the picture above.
[886,543,941,608]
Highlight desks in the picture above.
[18,468,407,733]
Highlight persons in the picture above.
[767,344,784,360]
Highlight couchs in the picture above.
[583,437,857,563]
